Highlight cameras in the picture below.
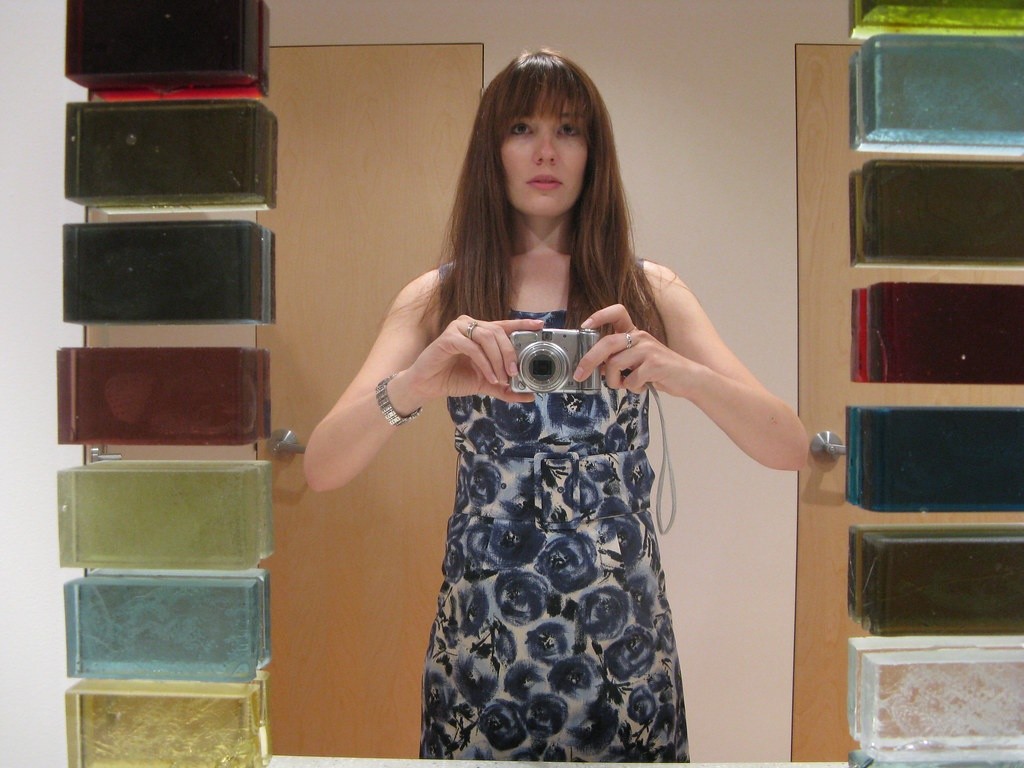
[508,328,602,393]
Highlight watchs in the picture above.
[375,374,422,427]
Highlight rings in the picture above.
[625,333,632,349]
[466,321,478,339]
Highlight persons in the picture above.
[304,51,810,763]
